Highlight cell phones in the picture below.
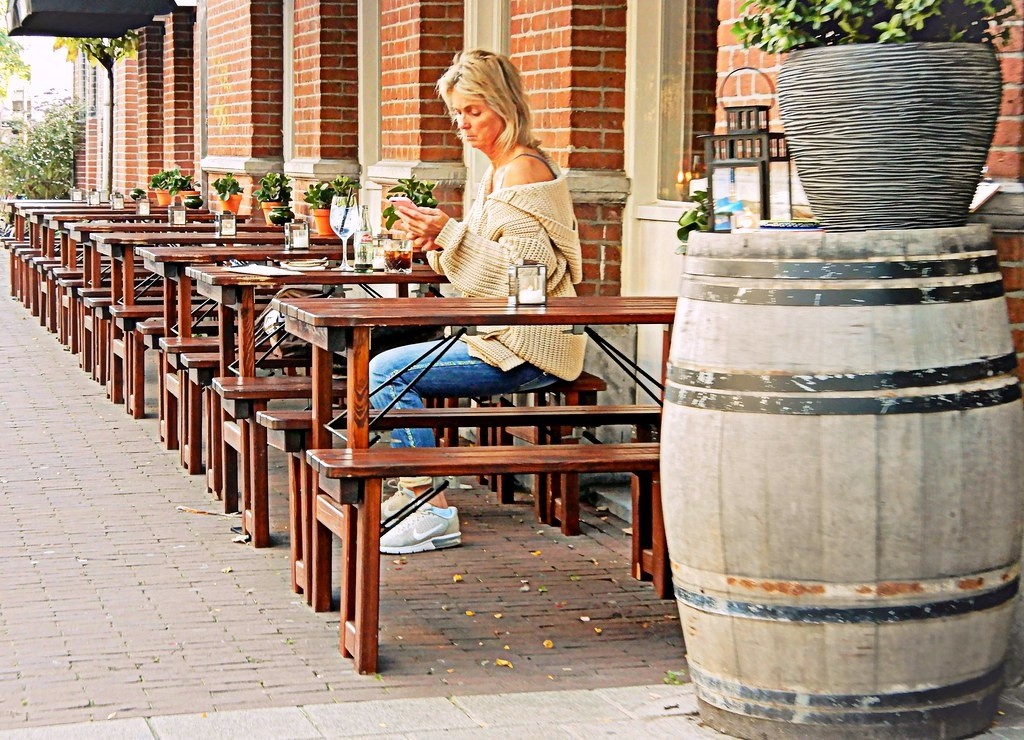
[389,196,422,218]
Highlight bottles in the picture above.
[268,206,295,226]
[184,195,203,210]
[354,205,374,273]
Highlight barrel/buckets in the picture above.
[659,222,1023,740]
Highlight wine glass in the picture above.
[329,195,359,272]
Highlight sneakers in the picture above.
[380,503,462,553]
[380,479,417,524]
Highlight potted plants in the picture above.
[252,173,292,225]
[169,169,202,208]
[149,170,172,206]
[733,0,1019,234]
[303,182,334,236]
[386,178,440,249]
[212,172,244,215]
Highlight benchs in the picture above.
[2,235,671,676]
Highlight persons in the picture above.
[369,51,586,552]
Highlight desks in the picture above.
[133,245,356,452]
[88,233,338,404]
[1,199,87,296]
[15,203,172,302]
[44,214,252,335]
[268,296,681,656]
[26,209,210,317]
[181,265,448,535]
[63,222,285,355]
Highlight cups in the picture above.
[382,240,413,274]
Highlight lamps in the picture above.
[692,63,793,237]
